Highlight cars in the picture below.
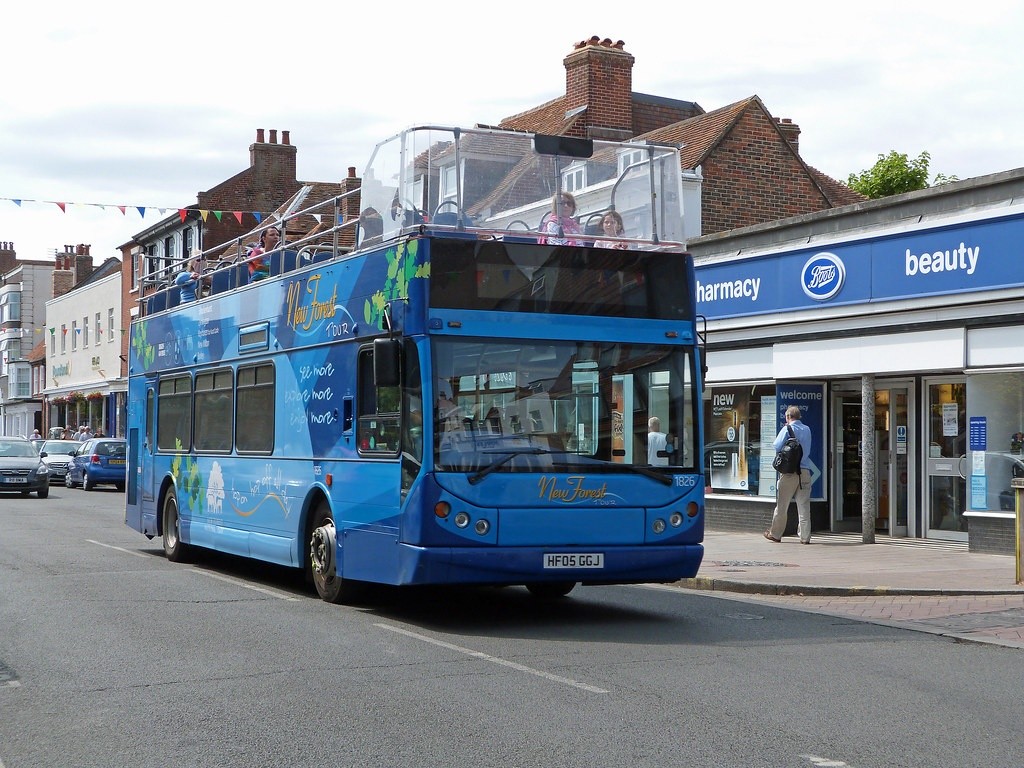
[705,440,760,470]
[0,435,51,499]
[38,439,84,482]
[30,439,47,453]
[65,438,126,491]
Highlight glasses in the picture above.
[193,258,200,262]
[560,200,574,207]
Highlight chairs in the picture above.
[144,240,347,316]
[416,200,604,237]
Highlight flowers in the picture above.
[86,391,104,401]
[66,391,75,401]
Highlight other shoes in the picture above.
[764,531,781,542]
[801,537,809,544]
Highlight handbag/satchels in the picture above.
[772,425,803,475]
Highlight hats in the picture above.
[79,426,85,429]
[84,425,91,430]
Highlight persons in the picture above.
[647,417,669,466]
[74,426,84,440]
[62,425,75,440]
[30,429,41,439]
[79,426,93,441]
[763,407,811,545]
[540,192,583,246]
[247,227,281,281]
[403,371,456,460]
[595,211,629,250]
[94,428,105,437]
[176,258,212,303]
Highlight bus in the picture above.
[121,125,708,605]
